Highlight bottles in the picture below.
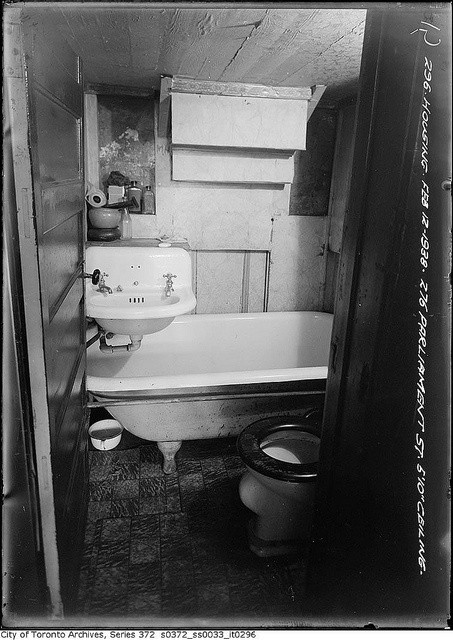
[128,180,143,212]
[121,193,133,241]
[141,185,156,214]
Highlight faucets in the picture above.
[95,274,113,295]
[161,273,175,298]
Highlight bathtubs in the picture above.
[83,310,342,474]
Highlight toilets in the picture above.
[237,411,333,557]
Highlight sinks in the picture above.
[86,289,180,308]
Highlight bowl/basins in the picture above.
[87,418,124,451]
